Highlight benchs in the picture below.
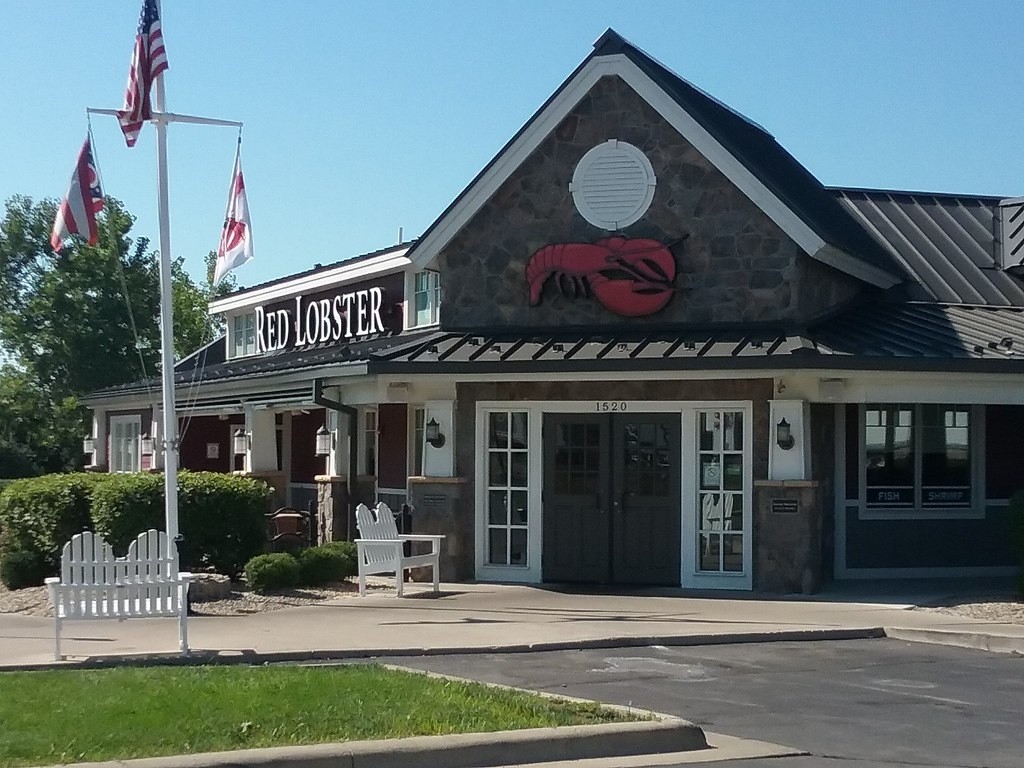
[44,529,197,659]
[354,502,446,598]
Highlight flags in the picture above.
[51,132,105,254]
[116,1,169,147]
[213,147,255,286]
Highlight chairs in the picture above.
[265,507,311,551]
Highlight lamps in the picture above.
[141,432,156,456]
[233,428,252,455]
[315,424,336,457]
[777,418,794,450]
[83,435,96,456]
[425,417,445,448]
